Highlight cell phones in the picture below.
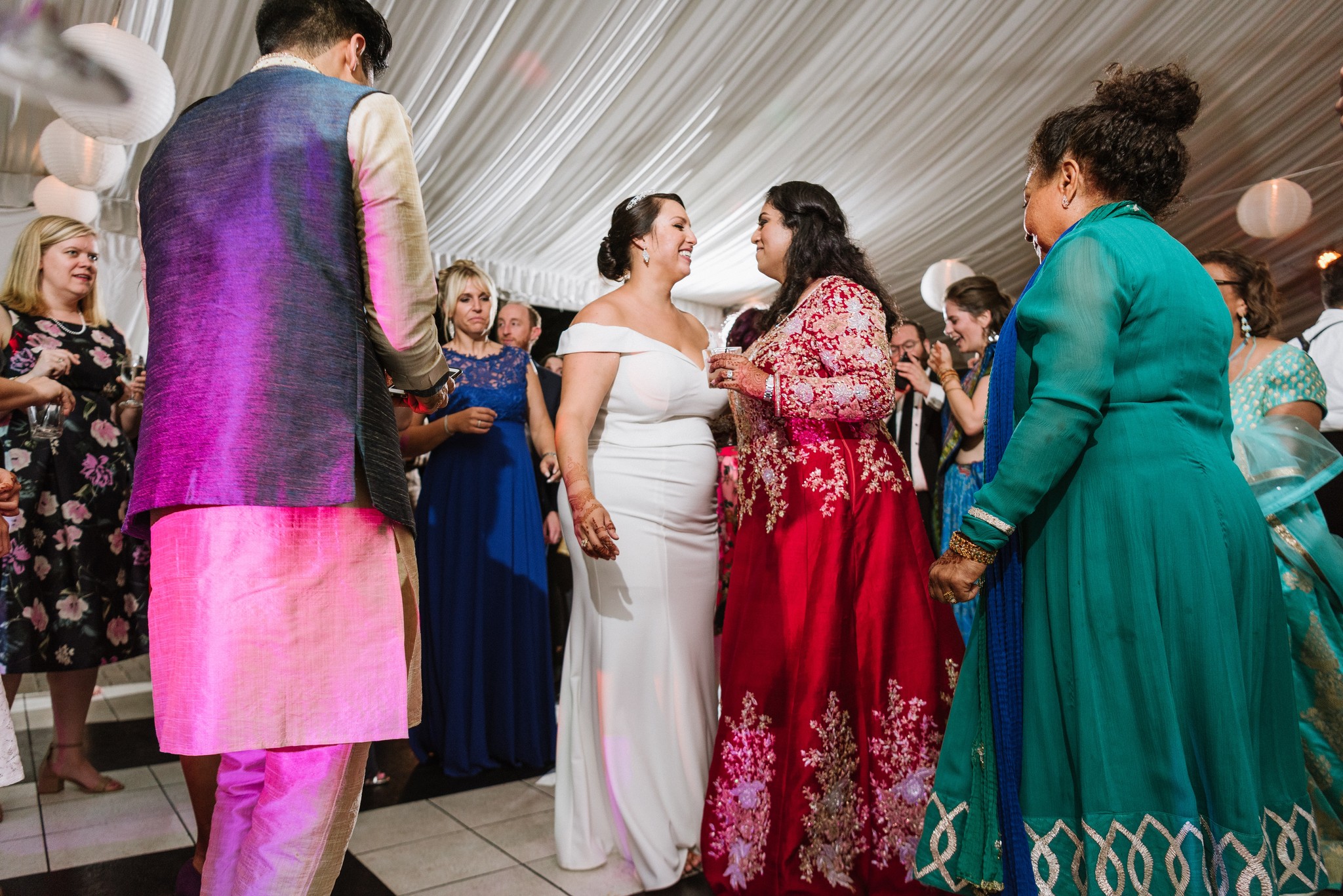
[894,352,919,390]
[388,366,464,398]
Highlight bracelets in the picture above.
[949,531,997,565]
[968,506,1014,536]
[937,367,962,392]
[444,414,453,436]
[542,453,556,457]
[8,376,17,380]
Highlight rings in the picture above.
[944,591,956,604]
[727,370,733,379]
[56,357,63,363]
[581,539,589,547]
[477,420,481,427]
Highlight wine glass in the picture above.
[118,353,145,409]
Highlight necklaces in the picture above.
[48,309,87,335]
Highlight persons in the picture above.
[913,61,1342,896]
[925,277,1016,647]
[700,181,966,896]
[0,215,147,794]
[711,308,776,634]
[882,319,948,524]
[370,260,564,792]
[125,0,463,896]
[553,189,770,891]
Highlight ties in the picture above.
[898,384,915,476]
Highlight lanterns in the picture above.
[1237,178,1312,238]
[920,259,976,314]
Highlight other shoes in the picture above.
[365,771,392,784]
[176,858,202,895]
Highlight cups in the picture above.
[26,399,65,440]
[701,346,742,388]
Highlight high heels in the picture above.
[38,742,125,794]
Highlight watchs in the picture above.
[764,374,774,402]
